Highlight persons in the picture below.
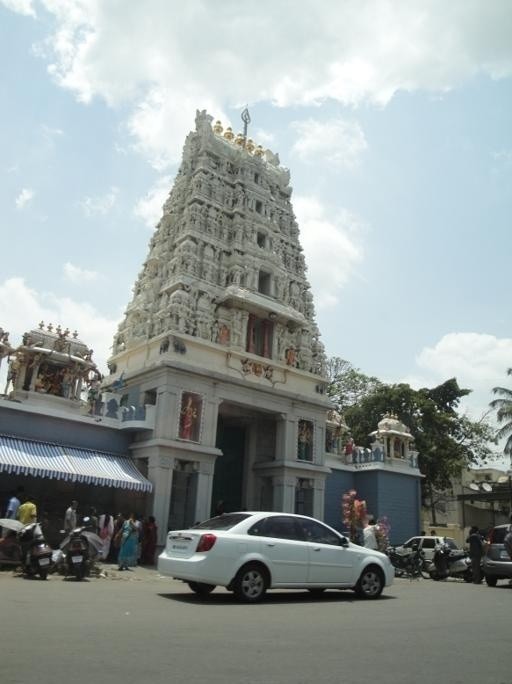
[299,423,311,459]
[210,320,220,343]
[0,490,159,571]
[217,324,229,343]
[287,344,294,364]
[0,320,102,415]
[326,433,401,464]
[420,512,512,587]
[362,520,381,550]
[181,395,198,439]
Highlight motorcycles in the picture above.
[59,513,105,583]
[15,523,56,581]
[428,537,484,584]
[385,543,434,579]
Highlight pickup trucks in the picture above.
[386,535,463,562]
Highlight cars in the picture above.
[158,511,396,603]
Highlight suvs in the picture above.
[479,523,511,588]
